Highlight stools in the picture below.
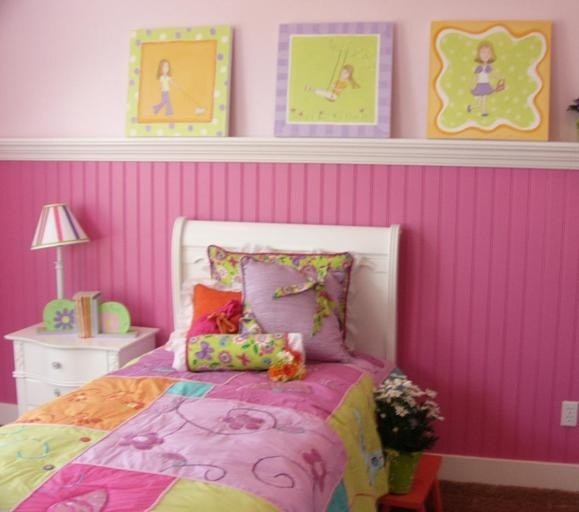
[378,454,444,512]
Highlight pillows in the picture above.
[169,242,363,373]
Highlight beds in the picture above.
[0,215,402,512]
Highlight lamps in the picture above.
[30,203,140,340]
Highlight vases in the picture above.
[385,448,421,495]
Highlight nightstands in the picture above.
[3,317,161,419]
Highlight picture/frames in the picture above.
[123,24,235,138]
[273,20,395,140]
[424,18,554,143]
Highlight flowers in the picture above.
[373,373,444,453]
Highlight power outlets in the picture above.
[559,400,578,428]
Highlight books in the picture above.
[75,291,101,339]
